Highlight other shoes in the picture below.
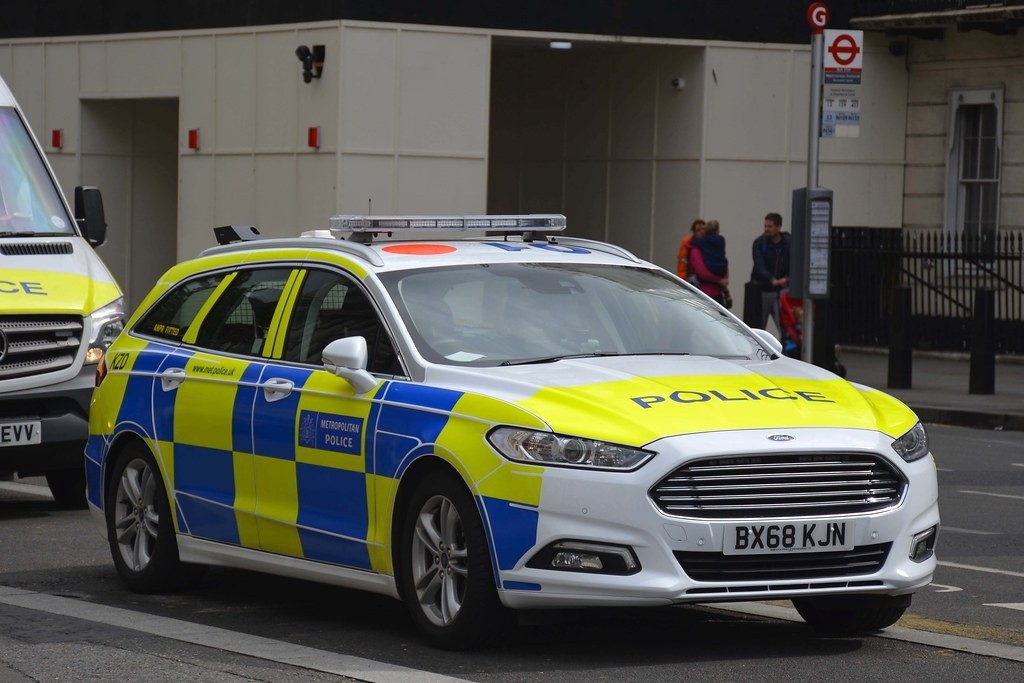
[835,344,840,359]
[723,296,733,309]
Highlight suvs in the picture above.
[81,212,941,650]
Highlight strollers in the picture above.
[772,281,847,377]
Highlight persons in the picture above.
[676,219,733,309]
[750,213,791,335]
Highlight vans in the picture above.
[1,77,127,509]
